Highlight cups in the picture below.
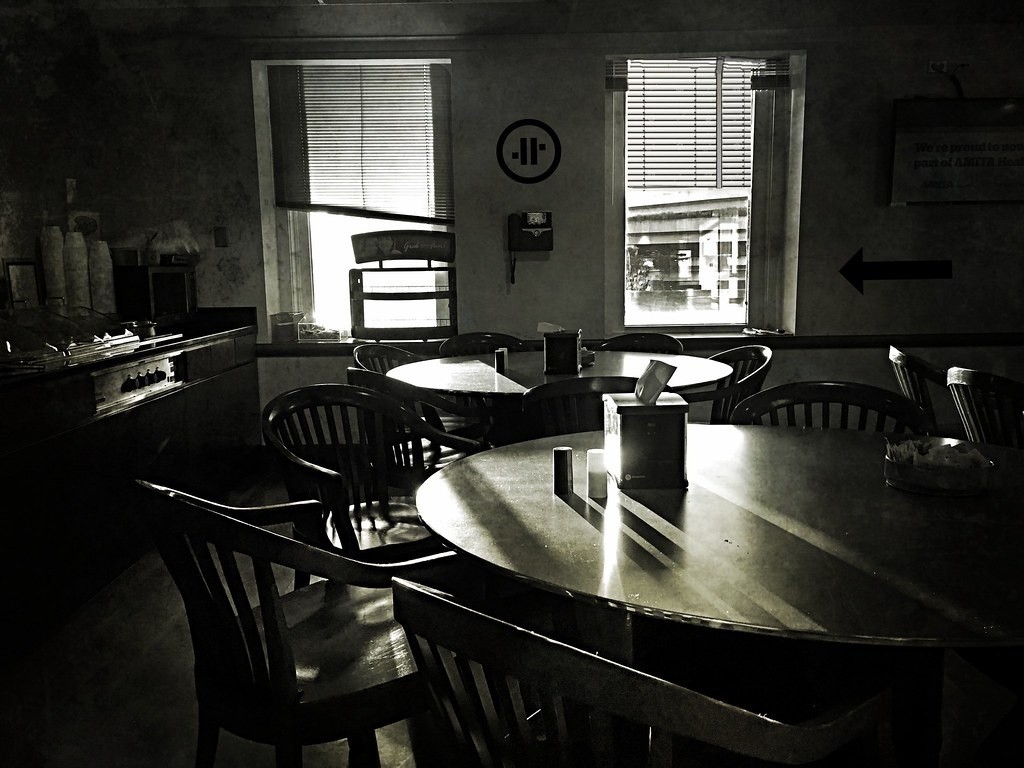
[39,226,115,318]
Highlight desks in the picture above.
[416,418,1022,768]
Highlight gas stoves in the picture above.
[0,332,183,414]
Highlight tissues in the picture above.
[537,321,583,375]
[602,358,692,491]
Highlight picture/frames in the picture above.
[2,258,49,311]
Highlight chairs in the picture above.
[390,574,902,768]
[134,477,475,767]
[344,330,1023,504]
[260,381,495,591]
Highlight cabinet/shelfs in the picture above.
[0,307,265,669]
